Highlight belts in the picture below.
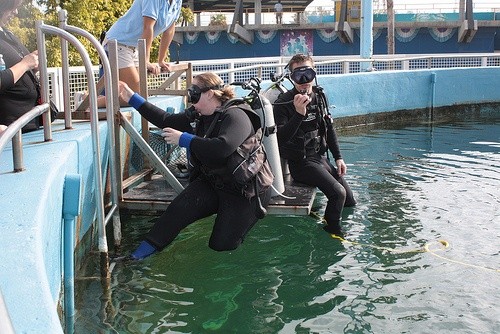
[104,37,136,49]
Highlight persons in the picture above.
[273,53,357,240]
[274,0,283,24]
[73,0,182,112]
[113,71,274,261]
[0,0,49,135]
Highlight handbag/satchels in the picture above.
[37,96,59,126]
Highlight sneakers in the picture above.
[74,92,86,111]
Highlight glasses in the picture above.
[290,66,316,84]
[187,84,225,104]
